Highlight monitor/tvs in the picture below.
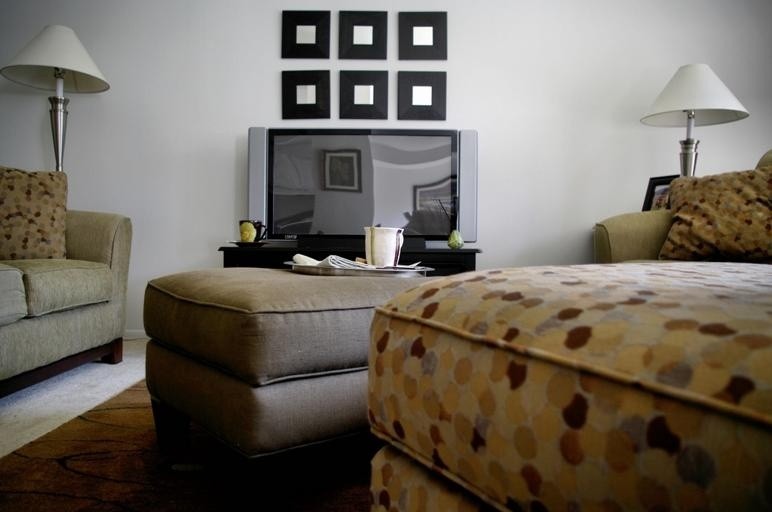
[247,127,478,249]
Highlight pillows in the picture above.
[657,166,772,261]
[0,166,69,260]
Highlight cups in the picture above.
[238,220,266,241]
[364,226,404,268]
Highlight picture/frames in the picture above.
[642,174,680,210]
[321,149,362,192]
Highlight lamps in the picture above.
[0,22,110,171]
[640,62,749,176]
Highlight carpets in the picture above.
[0,365,414,511]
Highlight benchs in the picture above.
[366,261,772,511]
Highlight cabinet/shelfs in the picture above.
[216,237,481,276]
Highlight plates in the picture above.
[229,241,272,248]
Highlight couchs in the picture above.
[0,207,133,399]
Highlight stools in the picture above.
[143,267,439,479]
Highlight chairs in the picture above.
[593,149,772,263]
